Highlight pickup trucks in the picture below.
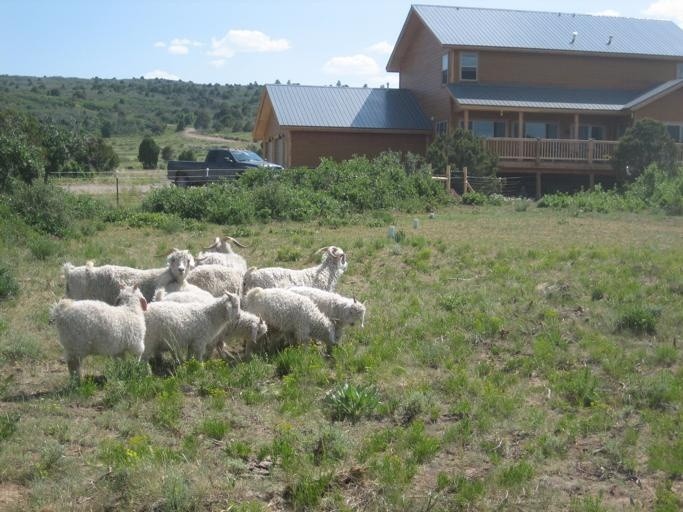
[168,148,284,189]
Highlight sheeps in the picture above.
[48,236,367,389]
